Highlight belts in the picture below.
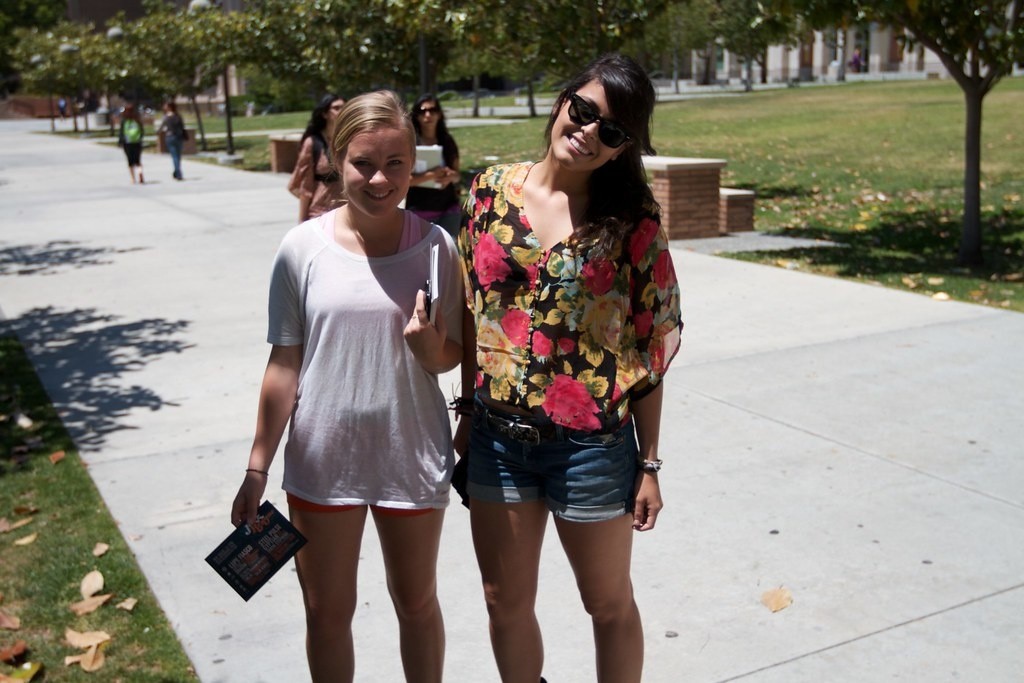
[487,414,618,447]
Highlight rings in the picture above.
[412,315,419,318]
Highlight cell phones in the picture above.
[425,280,432,320]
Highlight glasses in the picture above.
[419,107,438,115]
[568,92,631,148]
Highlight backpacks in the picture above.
[123,120,141,143]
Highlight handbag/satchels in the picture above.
[174,128,188,141]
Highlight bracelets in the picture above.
[637,452,663,473]
[244,468,268,476]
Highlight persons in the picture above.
[447,55,684,683]
[230,89,470,683]
[157,101,186,181]
[117,102,146,183]
[404,94,463,224]
[285,94,349,223]
